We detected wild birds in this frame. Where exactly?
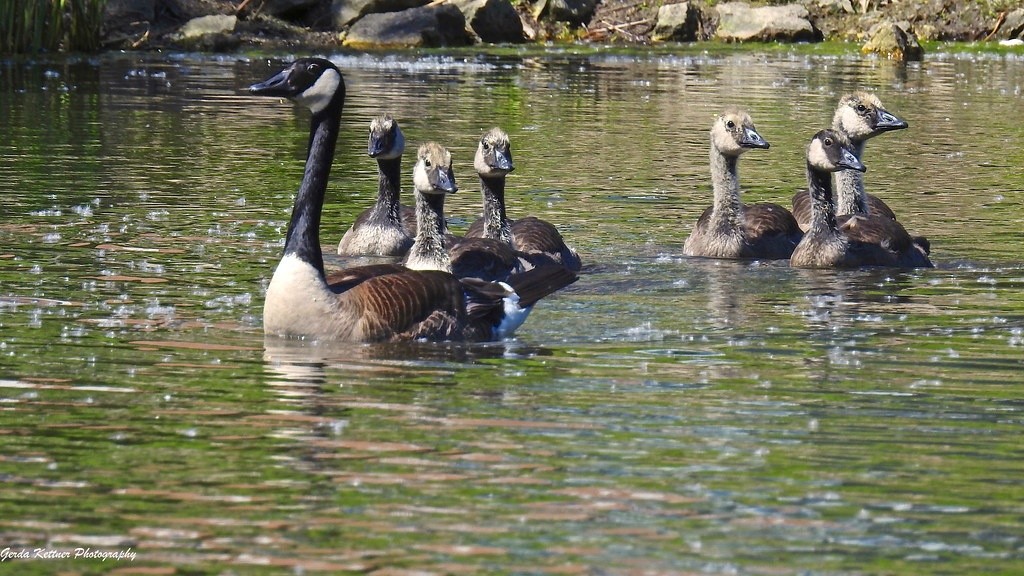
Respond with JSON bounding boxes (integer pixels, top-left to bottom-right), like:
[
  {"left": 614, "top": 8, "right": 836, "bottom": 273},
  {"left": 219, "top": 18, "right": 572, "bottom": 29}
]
[
  {"left": 682, "top": 89, "right": 937, "bottom": 270},
  {"left": 245, "top": 55, "right": 585, "bottom": 346}
]
